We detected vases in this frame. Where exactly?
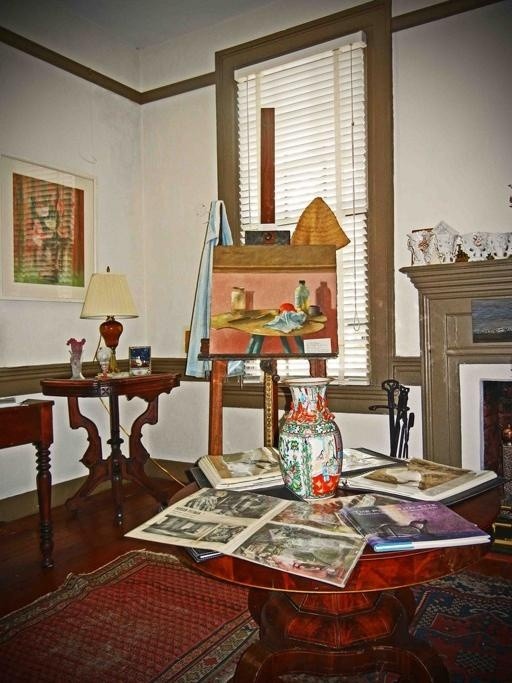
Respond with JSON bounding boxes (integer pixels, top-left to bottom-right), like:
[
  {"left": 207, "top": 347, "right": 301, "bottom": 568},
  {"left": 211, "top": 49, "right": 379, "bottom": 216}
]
[{"left": 278, "top": 377, "right": 344, "bottom": 502}]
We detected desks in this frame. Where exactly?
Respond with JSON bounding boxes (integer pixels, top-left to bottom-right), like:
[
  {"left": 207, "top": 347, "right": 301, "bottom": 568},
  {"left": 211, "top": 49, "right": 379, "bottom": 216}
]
[
  {"left": 167, "top": 479, "right": 502, "bottom": 682},
  {"left": 1, "top": 398, "right": 55, "bottom": 568},
  {"left": 39, "top": 372, "right": 183, "bottom": 526}
]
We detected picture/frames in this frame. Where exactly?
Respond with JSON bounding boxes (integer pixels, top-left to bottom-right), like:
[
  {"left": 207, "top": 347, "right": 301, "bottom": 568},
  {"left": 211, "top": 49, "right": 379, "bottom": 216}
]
[{"left": 1, "top": 152, "right": 98, "bottom": 303}]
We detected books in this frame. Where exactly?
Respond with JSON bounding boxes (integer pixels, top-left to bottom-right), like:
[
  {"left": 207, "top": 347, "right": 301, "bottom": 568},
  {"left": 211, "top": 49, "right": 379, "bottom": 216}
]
[
  {"left": 346, "top": 499, "right": 492, "bottom": 553},
  {"left": 309, "top": 493, "right": 414, "bottom": 507},
  {"left": 340, "top": 456, "right": 509, "bottom": 507},
  {"left": 188, "top": 444, "right": 396, "bottom": 491},
  {"left": 122, "top": 487, "right": 373, "bottom": 590},
  {"left": 157, "top": 504, "right": 222, "bottom": 563}
]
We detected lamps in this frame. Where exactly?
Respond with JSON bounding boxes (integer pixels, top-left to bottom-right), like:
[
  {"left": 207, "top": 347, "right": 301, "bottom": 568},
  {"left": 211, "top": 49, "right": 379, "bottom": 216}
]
[{"left": 80, "top": 266, "right": 140, "bottom": 376}]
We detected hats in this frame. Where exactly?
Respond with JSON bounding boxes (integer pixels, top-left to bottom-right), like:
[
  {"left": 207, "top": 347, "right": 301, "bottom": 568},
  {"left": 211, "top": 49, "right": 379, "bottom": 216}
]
[{"left": 289, "top": 197, "right": 351, "bottom": 249}]
